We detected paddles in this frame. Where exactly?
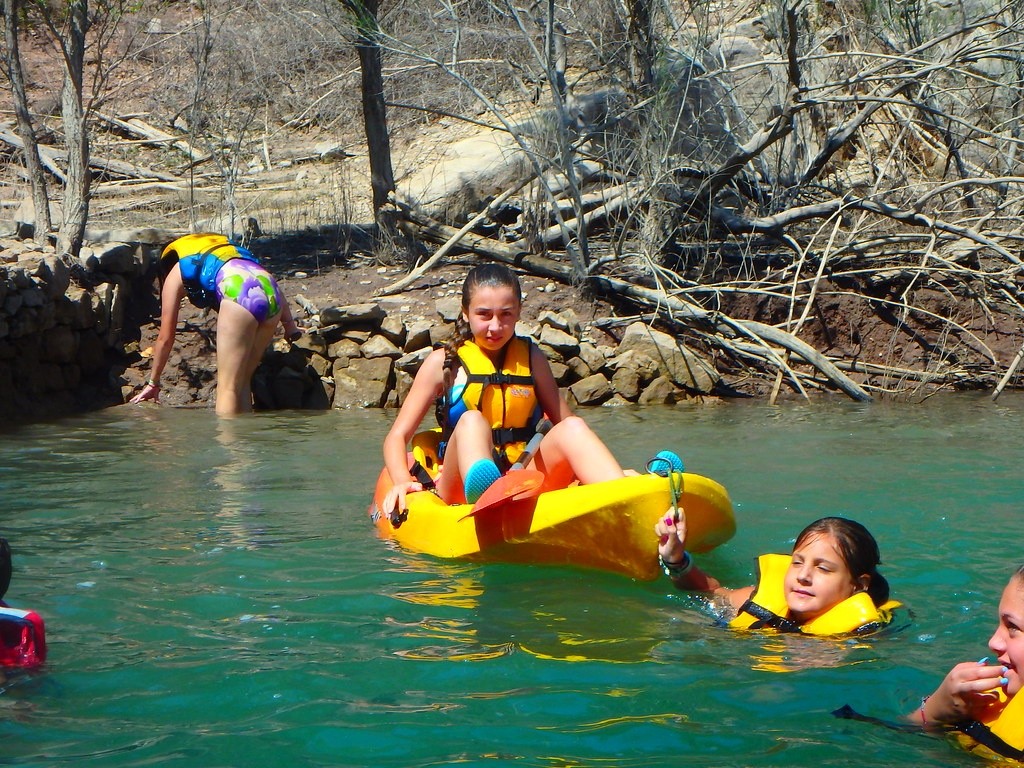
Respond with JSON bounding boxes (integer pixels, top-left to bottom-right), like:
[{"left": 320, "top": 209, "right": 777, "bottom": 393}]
[{"left": 456, "top": 418, "right": 552, "bottom": 526}]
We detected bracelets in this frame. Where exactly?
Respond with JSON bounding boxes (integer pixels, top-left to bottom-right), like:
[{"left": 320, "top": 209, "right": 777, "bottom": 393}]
[
  {"left": 658, "top": 551, "right": 695, "bottom": 578},
  {"left": 148, "top": 379, "right": 160, "bottom": 389},
  {"left": 279, "top": 316, "right": 293, "bottom": 326},
  {"left": 920, "top": 695, "right": 941, "bottom": 727}
]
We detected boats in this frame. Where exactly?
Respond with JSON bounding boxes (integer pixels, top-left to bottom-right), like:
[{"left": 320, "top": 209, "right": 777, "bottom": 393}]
[{"left": 370, "top": 428, "right": 739, "bottom": 580}]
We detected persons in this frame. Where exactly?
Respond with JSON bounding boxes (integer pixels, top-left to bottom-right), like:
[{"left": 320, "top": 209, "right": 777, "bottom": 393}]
[
  {"left": 382, "top": 263, "right": 628, "bottom": 514},
  {"left": 128, "top": 231, "right": 305, "bottom": 419},
  {"left": 654, "top": 505, "right": 893, "bottom": 639},
  {"left": 905, "top": 566, "right": 1023, "bottom": 767}
]
